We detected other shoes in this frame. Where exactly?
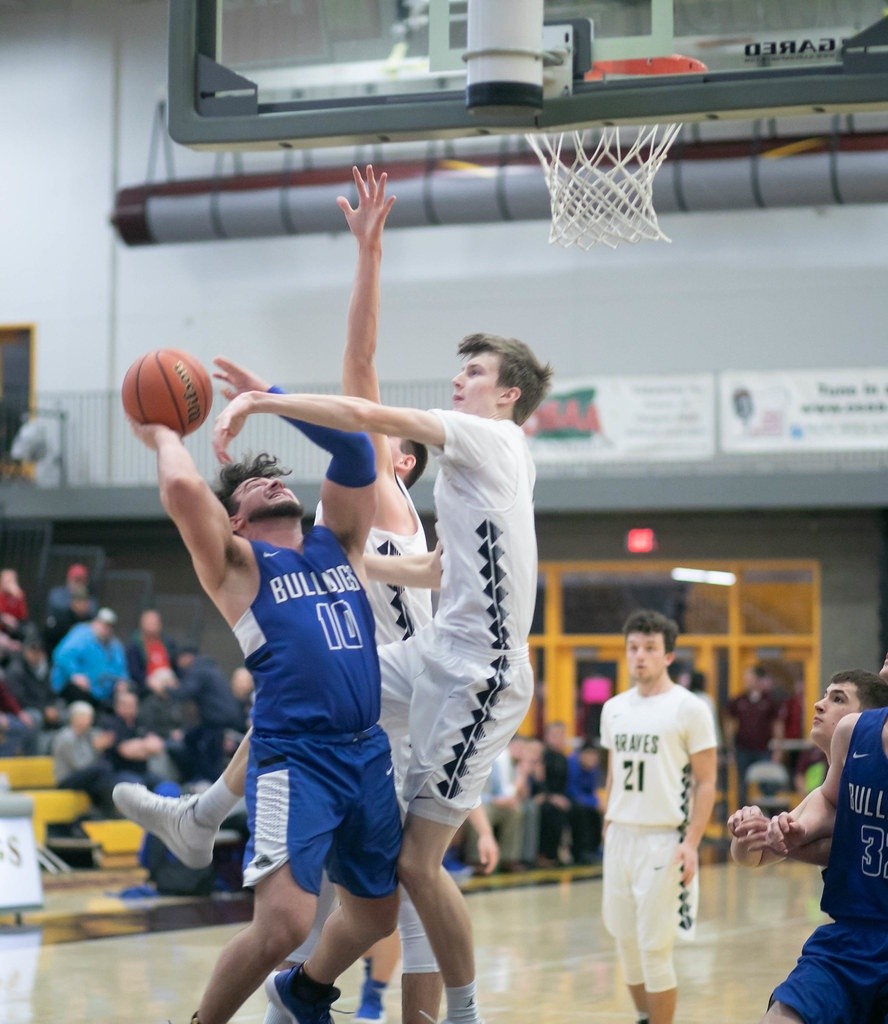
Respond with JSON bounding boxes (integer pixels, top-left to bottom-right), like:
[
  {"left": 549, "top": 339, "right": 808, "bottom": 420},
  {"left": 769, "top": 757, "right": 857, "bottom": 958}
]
[
  {"left": 440, "top": 855, "right": 477, "bottom": 877},
  {"left": 355, "top": 981, "right": 388, "bottom": 1022}
]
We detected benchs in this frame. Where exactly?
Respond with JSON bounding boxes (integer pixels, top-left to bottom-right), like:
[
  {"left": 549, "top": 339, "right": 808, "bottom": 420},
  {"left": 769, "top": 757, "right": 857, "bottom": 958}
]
[{"left": 0, "top": 731, "right": 240, "bottom": 891}]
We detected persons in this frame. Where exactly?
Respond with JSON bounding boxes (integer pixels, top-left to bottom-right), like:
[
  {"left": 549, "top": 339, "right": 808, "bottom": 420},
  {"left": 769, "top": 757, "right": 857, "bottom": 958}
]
[
  {"left": 600, "top": 609, "right": 719, "bottom": 1024},
  {"left": 268, "top": 166, "right": 524, "bottom": 1023},
  {"left": 137, "top": 355, "right": 408, "bottom": 1023},
  {"left": 727, "top": 654, "right": 888, "bottom": 1024},
  {"left": 113, "top": 332, "right": 552, "bottom": 1024},
  {"left": 729, "top": 667, "right": 791, "bottom": 820},
  {"left": 1, "top": 566, "right": 607, "bottom": 900}
]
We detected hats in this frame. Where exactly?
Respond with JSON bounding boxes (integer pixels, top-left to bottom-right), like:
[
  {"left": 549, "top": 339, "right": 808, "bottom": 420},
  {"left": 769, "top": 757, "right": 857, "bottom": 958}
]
[
  {"left": 98, "top": 608, "right": 117, "bottom": 625},
  {"left": 66, "top": 564, "right": 87, "bottom": 581}
]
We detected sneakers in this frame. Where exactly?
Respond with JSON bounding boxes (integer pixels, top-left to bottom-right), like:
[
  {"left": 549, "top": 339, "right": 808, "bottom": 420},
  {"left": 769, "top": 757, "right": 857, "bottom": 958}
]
[
  {"left": 112, "top": 782, "right": 218, "bottom": 868},
  {"left": 264, "top": 960, "right": 341, "bottom": 1024}
]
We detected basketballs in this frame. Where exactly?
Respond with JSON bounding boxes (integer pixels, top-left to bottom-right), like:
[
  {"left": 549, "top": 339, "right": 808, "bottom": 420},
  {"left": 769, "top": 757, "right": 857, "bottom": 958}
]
[{"left": 120, "top": 345, "right": 216, "bottom": 439}]
